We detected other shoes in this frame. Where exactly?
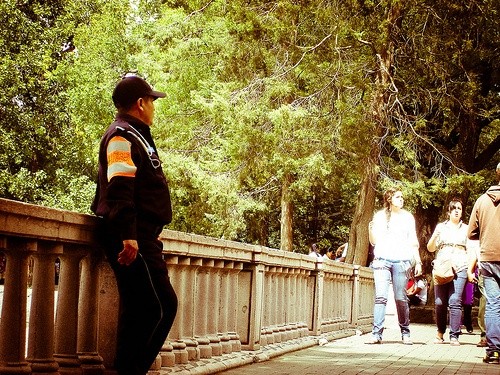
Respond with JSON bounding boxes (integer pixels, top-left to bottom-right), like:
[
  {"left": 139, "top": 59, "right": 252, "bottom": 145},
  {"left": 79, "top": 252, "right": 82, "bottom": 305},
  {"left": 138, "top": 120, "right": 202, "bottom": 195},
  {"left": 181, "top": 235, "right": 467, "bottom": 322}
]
[
  {"left": 364, "top": 337, "right": 380, "bottom": 344},
  {"left": 402, "top": 334, "right": 412, "bottom": 344},
  {"left": 436, "top": 337, "right": 444, "bottom": 343},
  {"left": 483, "top": 354, "right": 498, "bottom": 363},
  {"left": 469, "top": 330, "right": 472, "bottom": 334},
  {"left": 450, "top": 335, "right": 460, "bottom": 346},
  {"left": 477, "top": 339, "right": 488, "bottom": 346}
]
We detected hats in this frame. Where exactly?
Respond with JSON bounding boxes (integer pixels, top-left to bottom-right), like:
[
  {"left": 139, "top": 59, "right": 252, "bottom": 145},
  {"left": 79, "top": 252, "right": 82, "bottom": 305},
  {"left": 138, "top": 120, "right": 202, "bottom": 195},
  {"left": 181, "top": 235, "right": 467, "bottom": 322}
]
[
  {"left": 112, "top": 77, "right": 165, "bottom": 101},
  {"left": 311, "top": 244, "right": 319, "bottom": 250}
]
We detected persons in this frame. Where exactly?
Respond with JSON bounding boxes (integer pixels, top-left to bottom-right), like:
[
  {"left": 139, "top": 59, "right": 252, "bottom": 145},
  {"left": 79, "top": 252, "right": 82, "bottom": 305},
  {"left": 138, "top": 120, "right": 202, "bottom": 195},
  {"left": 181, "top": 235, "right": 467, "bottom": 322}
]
[
  {"left": 406, "top": 267, "right": 427, "bottom": 321},
  {"left": 426, "top": 198, "right": 469, "bottom": 346},
  {"left": 363, "top": 186, "right": 429, "bottom": 344},
  {"left": 467, "top": 173, "right": 500, "bottom": 365},
  {"left": 461, "top": 222, "right": 489, "bottom": 346},
  {"left": 90, "top": 76, "right": 178, "bottom": 375},
  {"left": 308, "top": 242, "right": 349, "bottom": 262}
]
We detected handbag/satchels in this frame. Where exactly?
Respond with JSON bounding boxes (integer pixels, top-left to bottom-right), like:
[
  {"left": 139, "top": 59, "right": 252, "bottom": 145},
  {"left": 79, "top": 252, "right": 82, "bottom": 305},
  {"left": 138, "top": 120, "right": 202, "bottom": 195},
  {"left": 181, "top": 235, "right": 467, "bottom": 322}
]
[{"left": 433, "top": 263, "right": 454, "bottom": 285}]
